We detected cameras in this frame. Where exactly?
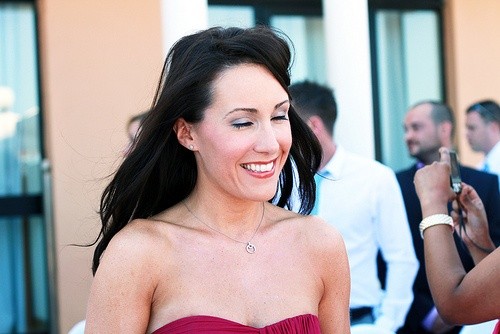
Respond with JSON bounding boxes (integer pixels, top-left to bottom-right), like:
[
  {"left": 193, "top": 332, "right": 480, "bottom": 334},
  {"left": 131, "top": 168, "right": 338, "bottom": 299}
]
[{"left": 439, "top": 152, "right": 462, "bottom": 194}]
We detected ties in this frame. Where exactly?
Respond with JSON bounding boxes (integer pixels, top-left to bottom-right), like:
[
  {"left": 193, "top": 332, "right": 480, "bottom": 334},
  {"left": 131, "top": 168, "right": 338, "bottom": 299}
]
[{"left": 310, "top": 171, "right": 329, "bottom": 216}]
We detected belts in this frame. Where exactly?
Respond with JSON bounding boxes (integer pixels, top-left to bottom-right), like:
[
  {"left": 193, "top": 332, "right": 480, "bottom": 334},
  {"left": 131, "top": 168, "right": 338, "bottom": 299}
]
[{"left": 350, "top": 306, "right": 372, "bottom": 326}]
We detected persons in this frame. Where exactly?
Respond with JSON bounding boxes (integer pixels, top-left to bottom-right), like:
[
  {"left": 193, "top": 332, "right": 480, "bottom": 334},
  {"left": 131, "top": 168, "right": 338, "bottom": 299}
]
[
  {"left": 271, "top": 80, "right": 420, "bottom": 334},
  {"left": 66, "top": 23, "right": 351, "bottom": 334}
]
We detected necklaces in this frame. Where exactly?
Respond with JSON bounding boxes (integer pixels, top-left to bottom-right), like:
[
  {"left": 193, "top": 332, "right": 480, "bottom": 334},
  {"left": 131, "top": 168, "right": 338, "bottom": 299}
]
[
  {"left": 376, "top": 101, "right": 500, "bottom": 333},
  {"left": 183, "top": 198, "right": 265, "bottom": 254}
]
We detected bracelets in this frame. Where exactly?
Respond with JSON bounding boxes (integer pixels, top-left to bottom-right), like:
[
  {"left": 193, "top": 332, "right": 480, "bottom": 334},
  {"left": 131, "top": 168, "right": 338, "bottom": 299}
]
[{"left": 420, "top": 213, "right": 453, "bottom": 240}]
